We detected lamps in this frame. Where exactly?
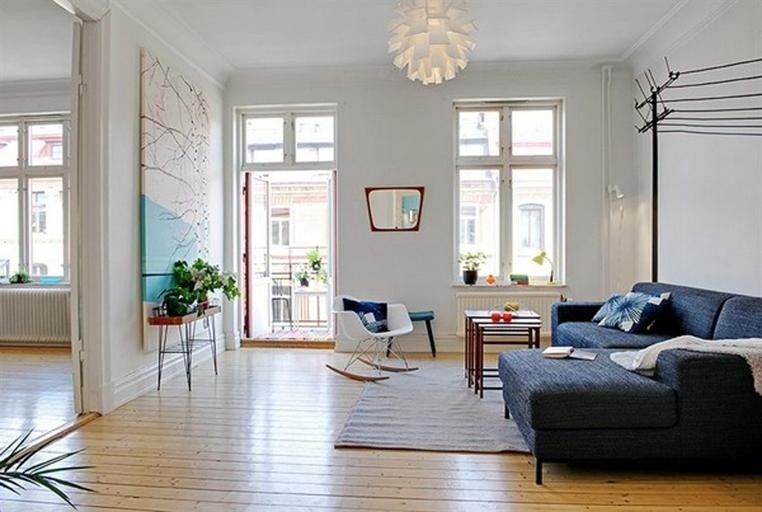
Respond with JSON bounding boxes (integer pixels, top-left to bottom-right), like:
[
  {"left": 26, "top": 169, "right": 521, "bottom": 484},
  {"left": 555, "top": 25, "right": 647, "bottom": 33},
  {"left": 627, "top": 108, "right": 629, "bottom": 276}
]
[
  {"left": 388, "top": 0, "right": 478, "bottom": 87},
  {"left": 532, "top": 250, "right": 555, "bottom": 284}
]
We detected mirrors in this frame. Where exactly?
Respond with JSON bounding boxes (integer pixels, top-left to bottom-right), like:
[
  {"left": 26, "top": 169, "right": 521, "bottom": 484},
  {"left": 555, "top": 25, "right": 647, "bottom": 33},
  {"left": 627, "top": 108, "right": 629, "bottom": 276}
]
[{"left": 364, "top": 186, "right": 425, "bottom": 232}]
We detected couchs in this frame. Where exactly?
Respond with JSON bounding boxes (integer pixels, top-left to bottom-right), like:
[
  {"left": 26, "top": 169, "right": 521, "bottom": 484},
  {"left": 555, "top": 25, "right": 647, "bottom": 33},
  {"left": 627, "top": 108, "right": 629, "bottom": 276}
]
[{"left": 550, "top": 282, "right": 762, "bottom": 474}]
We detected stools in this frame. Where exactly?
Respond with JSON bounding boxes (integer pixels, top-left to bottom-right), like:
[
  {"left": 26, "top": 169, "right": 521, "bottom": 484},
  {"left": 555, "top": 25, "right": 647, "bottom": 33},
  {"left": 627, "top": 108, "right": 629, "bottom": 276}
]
[
  {"left": 386, "top": 310, "right": 437, "bottom": 358},
  {"left": 495, "top": 347, "right": 678, "bottom": 488},
  {"left": 272, "top": 294, "right": 292, "bottom": 330}
]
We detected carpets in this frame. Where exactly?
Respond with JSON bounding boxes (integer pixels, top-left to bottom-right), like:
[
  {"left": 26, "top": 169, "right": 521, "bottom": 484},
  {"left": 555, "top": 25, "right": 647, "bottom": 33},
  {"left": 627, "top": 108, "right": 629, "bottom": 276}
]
[{"left": 335, "top": 356, "right": 532, "bottom": 456}]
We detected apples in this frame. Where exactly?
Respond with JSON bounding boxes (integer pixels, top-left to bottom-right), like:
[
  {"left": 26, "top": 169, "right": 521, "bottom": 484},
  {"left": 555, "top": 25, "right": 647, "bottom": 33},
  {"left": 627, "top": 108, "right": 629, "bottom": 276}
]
[
  {"left": 491, "top": 313, "right": 500, "bottom": 321},
  {"left": 502, "top": 313, "right": 513, "bottom": 322}
]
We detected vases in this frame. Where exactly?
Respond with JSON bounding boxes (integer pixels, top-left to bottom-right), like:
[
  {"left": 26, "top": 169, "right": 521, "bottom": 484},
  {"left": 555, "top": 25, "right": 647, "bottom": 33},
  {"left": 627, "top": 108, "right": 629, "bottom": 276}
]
[{"left": 195, "top": 300, "right": 209, "bottom": 310}]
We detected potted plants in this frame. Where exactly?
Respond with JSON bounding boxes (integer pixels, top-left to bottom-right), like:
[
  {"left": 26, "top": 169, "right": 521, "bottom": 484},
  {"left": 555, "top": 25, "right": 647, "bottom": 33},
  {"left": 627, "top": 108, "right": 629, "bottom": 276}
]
[
  {"left": 458, "top": 249, "right": 487, "bottom": 285},
  {"left": 294, "top": 250, "right": 322, "bottom": 287},
  {"left": 157, "top": 288, "right": 196, "bottom": 317}
]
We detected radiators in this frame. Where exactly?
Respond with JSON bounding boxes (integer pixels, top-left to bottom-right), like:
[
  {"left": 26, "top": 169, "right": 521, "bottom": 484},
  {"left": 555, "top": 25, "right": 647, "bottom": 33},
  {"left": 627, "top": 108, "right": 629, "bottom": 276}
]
[
  {"left": 455, "top": 291, "right": 563, "bottom": 339},
  {"left": 0, "top": 287, "right": 71, "bottom": 344}
]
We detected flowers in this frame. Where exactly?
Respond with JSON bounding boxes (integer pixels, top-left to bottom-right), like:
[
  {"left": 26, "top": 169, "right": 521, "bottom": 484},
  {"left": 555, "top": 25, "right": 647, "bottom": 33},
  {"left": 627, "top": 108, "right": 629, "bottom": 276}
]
[{"left": 173, "top": 258, "right": 241, "bottom": 302}]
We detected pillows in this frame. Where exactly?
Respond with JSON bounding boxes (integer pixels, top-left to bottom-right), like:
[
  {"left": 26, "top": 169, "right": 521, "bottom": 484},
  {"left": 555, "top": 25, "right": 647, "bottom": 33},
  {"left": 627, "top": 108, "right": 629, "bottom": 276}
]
[
  {"left": 591, "top": 290, "right": 672, "bottom": 335},
  {"left": 343, "top": 298, "right": 389, "bottom": 332}
]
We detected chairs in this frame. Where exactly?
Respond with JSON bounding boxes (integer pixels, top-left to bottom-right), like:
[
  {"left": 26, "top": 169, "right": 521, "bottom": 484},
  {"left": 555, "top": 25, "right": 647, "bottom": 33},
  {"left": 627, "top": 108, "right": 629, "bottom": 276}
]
[{"left": 326, "top": 294, "right": 419, "bottom": 380}]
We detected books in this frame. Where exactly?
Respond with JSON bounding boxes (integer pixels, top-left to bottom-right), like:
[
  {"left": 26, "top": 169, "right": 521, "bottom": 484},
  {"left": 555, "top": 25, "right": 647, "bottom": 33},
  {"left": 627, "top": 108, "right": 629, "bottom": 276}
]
[{"left": 541, "top": 345, "right": 598, "bottom": 360}]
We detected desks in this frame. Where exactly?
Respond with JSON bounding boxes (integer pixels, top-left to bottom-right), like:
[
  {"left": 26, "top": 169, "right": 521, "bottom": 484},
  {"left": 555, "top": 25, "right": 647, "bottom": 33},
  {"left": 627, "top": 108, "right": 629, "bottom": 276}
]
[
  {"left": 149, "top": 305, "right": 222, "bottom": 391},
  {"left": 294, "top": 287, "right": 329, "bottom": 332}
]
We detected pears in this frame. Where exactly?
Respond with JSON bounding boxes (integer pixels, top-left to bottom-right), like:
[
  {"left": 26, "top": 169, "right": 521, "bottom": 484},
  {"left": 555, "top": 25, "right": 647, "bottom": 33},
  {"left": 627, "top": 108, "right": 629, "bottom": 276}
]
[{"left": 504, "top": 302, "right": 520, "bottom": 312}]
